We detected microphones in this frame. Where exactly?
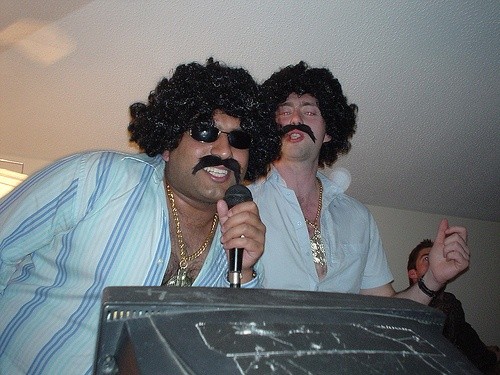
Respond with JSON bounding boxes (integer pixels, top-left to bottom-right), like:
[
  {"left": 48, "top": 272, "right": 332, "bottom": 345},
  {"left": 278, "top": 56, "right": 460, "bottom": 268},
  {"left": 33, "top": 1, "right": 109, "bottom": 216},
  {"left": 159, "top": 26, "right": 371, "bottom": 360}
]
[{"left": 222, "top": 184, "right": 251, "bottom": 289}]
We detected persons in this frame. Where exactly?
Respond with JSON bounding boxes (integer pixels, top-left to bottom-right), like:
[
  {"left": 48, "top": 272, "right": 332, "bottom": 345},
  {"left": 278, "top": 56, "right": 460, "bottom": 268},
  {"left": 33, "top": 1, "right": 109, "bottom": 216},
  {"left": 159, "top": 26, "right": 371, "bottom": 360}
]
[
  {"left": 406, "top": 237, "right": 468, "bottom": 322},
  {"left": 239, "top": 61, "right": 472, "bottom": 306},
  {"left": 1, "top": 59, "right": 283, "bottom": 374}
]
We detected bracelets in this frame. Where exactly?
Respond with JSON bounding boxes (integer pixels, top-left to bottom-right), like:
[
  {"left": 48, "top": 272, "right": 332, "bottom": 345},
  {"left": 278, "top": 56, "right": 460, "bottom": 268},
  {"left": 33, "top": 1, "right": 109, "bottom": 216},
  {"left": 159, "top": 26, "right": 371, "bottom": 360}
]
[{"left": 415, "top": 274, "right": 436, "bottom": 298}]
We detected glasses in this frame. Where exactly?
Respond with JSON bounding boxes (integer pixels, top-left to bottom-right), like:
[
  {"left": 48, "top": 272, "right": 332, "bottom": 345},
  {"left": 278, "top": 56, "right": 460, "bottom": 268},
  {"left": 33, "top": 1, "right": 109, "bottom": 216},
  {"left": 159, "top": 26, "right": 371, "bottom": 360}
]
[{"left": 189, "top": 122, "right": 251, "bottom": 149}]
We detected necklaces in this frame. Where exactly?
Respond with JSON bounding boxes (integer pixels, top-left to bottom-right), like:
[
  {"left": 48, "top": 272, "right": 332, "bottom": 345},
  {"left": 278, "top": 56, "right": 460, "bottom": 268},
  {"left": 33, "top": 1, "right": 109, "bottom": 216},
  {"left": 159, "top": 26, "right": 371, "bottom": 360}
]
[
  {"left": 163, "top": 183, "right": 217, "bottom": 287},
  {"left": 302, "top": 175, "right": 326, "bottom": 268}
]
